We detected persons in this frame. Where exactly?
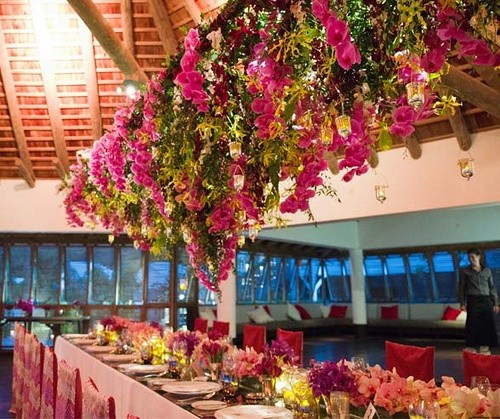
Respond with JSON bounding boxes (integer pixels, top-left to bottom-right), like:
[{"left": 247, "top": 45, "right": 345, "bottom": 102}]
[{"left": 459, "top": 248, "right": 498, "bottom": 354}]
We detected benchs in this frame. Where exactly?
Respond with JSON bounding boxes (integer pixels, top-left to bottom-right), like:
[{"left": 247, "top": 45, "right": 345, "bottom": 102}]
[
  {"left": 199, "top": 302, "right": 339, "bottom": 345},
  {"left": 329, "top": 303, "right": 468, "bottom": 344}
]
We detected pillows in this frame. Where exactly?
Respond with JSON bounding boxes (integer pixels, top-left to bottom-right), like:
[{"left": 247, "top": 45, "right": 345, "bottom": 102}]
[
  {"left": 287, "top": 303, "right": 313, "bottom": 321},
  {"left": 437, "top": 306, "right": 467, "bottom": 321},
  {"left": 381, "top": 305, "right": 399, "bottom": 320},
  {"left": 320, "top": 304, "right": 348, "bottom": 319},
  {"left": 246, "top": 306, "right": 274, "bottom": 324}
]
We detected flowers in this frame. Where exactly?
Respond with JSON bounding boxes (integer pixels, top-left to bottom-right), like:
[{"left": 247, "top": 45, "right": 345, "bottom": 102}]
[
  {"left": 92, "top": 316, "right": 500, "bottom": 419},
  {"left": 306, "top": 358, "right": 500, "bottom": 419},
  {"left": 64, "top": 0, "right": 500, "bottom": 298}
]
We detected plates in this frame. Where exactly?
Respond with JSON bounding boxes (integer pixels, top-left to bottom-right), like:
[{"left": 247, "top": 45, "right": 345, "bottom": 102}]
[
  {"left": 62, "top": 332, "right": 118, "bottom": 353},
  {"left": 191, "top": 400, "right": 227, "bottom": 410},
  {"left": 104, "top": 354, "right": 133, "bottom": 361},
  {"left": 214, "top": 405, "right": 294, "bottom": 419},
  {"left": 160, "top": 381, "right": 220, "bottom": 398},
  {"left": 150, "top": 377, "right": 176, "bottom": 385},
  {"left": 119, "top": 363, "right": 165, "bottom": 373}
]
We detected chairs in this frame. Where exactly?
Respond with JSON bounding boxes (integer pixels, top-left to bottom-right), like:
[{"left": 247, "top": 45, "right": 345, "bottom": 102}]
[
  {"left": 8, "top": 321, "right": 144, "bottom": 419},
  {"left": 194, "top": 317, "right": 500, "bottom": 393}
]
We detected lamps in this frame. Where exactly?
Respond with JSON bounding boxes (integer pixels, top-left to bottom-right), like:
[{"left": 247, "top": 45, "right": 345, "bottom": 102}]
[
  {"left": 375, "top": 185, "right": 389, "bottom": 204},
  {"left": 457, "top": 158, "right": 474, "bottom": 182}
]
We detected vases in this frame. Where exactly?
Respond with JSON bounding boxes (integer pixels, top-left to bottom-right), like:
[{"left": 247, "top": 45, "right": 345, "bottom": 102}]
[
  {"left": 220, "top": 369, "right": 239, "bottom": 397},
  {"left": 209, "top": 363, "right": 223, "bottom": 381},
  {"left": 169, "top": 355, "right": 182, "bottom": 375},
  {"left": 262, "top": 375, "right": 278, "bottom": 404},
  {"left": 330, "top": 391, "right": 349, "bottom": 419}
]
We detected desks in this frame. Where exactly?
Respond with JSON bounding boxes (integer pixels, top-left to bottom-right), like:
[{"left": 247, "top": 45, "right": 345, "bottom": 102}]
[{"left": 54, "top": 334, "right": 325, "bottom": 419}]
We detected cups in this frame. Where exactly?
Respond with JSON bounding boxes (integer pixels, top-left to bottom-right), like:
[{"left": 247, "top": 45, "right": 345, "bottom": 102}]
[
  {"left": 261, "top": 374, "right": 276, "bottom": 400},
  {"left": 167, "top": 356, "right": 179, "bottom": 378},
  {"left": 140, "top": 345, "right": 153, "bottom": 365},
  {"left": 408, "top": 397, "right": 439, "bottom": 419},
  {"left": 122, "top": 334, "right": 132, "bottom": 353},
  {"left": 293, "top": 393, "right": 319, "bottom": 419},
  {"left": 92, "top": 320, "right": 117, "bottom": 346},
  {"left": 221, "top": 371, "right": 238, "bottom": 406},
  {"left": 330, "top": 392, "right": 350, "bottom": 419},
  {"left": 470, "top": 375, "right": 491, "bottom": 397}
]
